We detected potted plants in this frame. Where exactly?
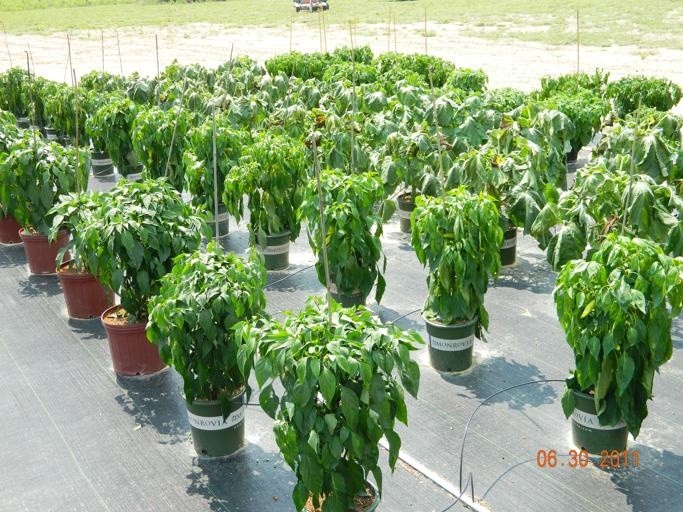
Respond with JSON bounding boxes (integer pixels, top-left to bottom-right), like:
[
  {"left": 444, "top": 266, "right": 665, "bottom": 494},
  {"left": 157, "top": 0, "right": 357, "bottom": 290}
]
[
  {"left": 552, "top": 227, "right": 683, "bottom": 458},
  {"left": 103, "top": 101, "right": 145, "bottom": 183},
  {"left": 44, "top": 190, "right": 122, "bottom": 321},
  {"left": 145, "top": 240, "right": 272, "bottom": 460},
  {"left": 543, "top": 86, "right": 610, "bottom": 163},
  {"left": 0, "top": 131, "right": 92, "bottom": 274},
  {"left": 8, "top": 70, "right": 30, "bottom": 129},
  {"left": 45, "top": 95, "right": 65, "bottom": 142},
  {"left": 368, "top": 87, "right": 457, "bottom": 234},
  {"left": 77, "top": 177, "right": 214, "bottom": 378},
  {"left": 230, "top": 295, "right": 424, "bottom": 508},
  {"left": 131, "top": 110, "right": 183, "bottom": 205},
  {"left": 85, "top": 112, "right": 115, "bottom": 178},
  {"left": 0, "top": 127, "right": 44, "bottom": 246},
  {"left": 410, "top": 185, "right": 503, "bottom": 374},
  {"left": 295, "top": 168, "right": 396, "bottom": 316},
  {"left": 182, "top": 115, "right": 246, "bottom": 240},
  {"left": 445, "top": 129, "right": 547, "bottom": 265},
  {"left": 222, "top": 135, "right": 310, "bottom": 271}
]
